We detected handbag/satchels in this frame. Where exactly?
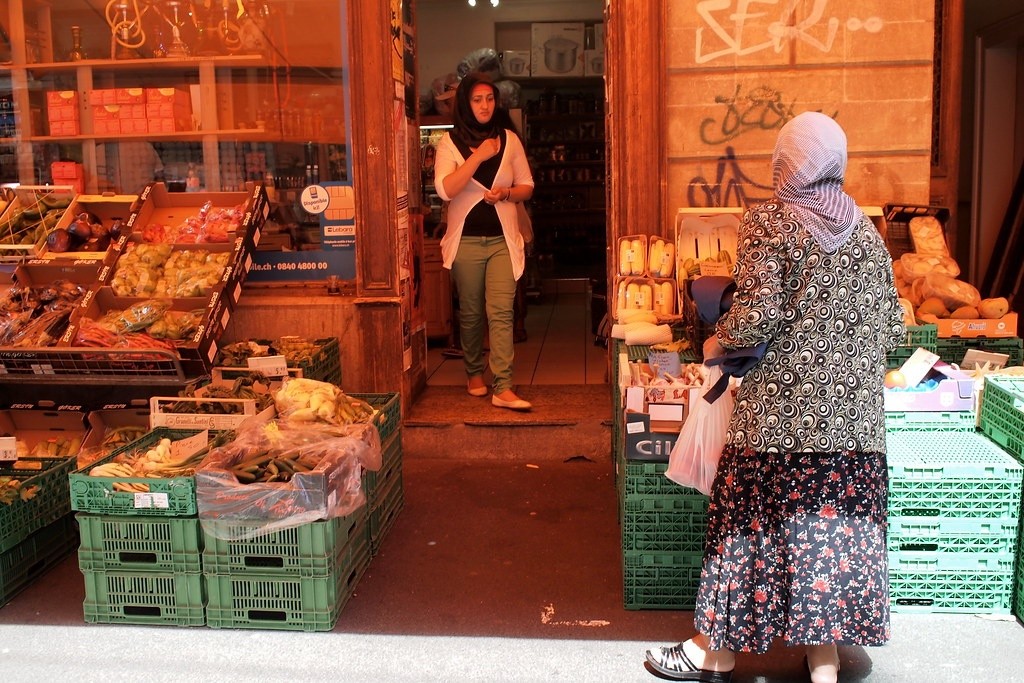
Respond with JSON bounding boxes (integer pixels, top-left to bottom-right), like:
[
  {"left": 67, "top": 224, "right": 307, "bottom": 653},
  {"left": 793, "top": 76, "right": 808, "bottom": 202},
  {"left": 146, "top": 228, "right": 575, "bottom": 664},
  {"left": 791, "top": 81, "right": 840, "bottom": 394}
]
[
  {"left": 691, "top": 276, "right": 770, "bottom": 404},
  {"left": 664, "top": 364, "right": 734, "bottom": 496}
]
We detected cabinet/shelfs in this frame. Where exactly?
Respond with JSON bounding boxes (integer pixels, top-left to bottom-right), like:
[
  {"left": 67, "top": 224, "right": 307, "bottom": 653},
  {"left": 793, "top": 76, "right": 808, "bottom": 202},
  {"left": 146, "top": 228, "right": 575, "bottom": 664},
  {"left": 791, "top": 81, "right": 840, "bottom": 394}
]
[
  {"left": 0, "top": 0, "right": 266, "bottom": 195},
  {"left": 504, "top": 77, "right": 606, "bottom": 216}
]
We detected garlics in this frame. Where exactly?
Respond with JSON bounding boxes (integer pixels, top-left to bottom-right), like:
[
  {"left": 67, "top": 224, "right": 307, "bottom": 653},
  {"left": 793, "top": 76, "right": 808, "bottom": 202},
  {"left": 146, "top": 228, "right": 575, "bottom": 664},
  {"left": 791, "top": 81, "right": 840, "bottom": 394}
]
[{"left": 623, "top": 359, "right": 703, "bottom": 386}]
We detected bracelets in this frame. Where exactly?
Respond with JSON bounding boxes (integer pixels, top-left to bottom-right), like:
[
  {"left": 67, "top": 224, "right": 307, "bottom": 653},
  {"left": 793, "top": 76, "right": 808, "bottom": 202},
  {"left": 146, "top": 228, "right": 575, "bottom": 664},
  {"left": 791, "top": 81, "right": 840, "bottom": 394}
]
[{"left": 506, "top": 188, "right": 510, "bottom": 200}]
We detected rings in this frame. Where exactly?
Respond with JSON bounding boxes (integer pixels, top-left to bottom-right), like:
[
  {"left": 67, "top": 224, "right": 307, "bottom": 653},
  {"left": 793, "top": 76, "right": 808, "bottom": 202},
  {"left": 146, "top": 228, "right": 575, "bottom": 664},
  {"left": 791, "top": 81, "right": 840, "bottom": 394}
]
[{"left": 487, "top": 201, "right": 490, "bottom": 203}]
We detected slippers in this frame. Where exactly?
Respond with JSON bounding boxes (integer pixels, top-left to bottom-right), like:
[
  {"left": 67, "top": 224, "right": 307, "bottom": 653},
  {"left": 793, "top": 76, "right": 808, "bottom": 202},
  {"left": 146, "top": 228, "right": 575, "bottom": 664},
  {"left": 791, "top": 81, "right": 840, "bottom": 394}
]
[
  {"left": 803, "top": 654, "right": 840, "bottom": 683},
  {"left": 645, "top": 639, "right": 735, "bottom": 683}
]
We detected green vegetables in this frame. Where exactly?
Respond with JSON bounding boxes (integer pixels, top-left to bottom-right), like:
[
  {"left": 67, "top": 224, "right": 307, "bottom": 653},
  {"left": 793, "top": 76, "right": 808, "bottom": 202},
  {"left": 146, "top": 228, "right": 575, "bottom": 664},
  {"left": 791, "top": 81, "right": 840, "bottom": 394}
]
[{"left": 157, "top": 338, "right": 279, "bottom": 414}]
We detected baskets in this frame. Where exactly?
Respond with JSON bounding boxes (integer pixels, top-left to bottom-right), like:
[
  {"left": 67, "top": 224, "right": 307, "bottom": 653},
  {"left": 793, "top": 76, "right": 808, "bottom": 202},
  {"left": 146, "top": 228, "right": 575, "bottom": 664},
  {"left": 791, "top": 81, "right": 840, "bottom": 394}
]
[
  {"left": 883, "top": 203, "right": 952, "bottom": 280},
  {"left": 68, "top": 425, "right": 236, "bottom": 515},
  {"left": 345, "top": 392, "right": 400, "bottom": 446}
]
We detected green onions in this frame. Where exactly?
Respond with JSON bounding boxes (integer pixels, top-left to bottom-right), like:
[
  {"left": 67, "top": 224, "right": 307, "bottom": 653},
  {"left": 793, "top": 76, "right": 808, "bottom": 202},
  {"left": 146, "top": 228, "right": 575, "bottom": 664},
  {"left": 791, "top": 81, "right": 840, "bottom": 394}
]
[{"left": 86, "top": 427, "right": 239, "bottom": 494}]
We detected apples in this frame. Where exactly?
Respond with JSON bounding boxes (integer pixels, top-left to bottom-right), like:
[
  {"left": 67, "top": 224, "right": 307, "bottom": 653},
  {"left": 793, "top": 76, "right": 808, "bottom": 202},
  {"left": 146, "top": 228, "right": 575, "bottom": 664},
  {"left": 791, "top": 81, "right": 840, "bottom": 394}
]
[{"left": 883, "top": 371, "right": 906, "bottom": 387}]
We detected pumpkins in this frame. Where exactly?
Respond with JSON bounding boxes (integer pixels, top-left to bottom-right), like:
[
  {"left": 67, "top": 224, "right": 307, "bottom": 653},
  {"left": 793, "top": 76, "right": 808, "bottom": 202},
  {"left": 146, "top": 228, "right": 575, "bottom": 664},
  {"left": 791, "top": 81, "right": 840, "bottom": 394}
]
[{"left": 918, "top": 294, "right": 1008, "bottom": 319}]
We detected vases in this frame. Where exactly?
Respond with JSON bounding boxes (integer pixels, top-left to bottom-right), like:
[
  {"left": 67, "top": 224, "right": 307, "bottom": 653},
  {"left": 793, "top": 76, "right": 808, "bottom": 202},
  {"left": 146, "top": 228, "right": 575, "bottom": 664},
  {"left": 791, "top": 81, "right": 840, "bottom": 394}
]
[{"left": 62, "top": 25, "right": 86, "bottom": 60}]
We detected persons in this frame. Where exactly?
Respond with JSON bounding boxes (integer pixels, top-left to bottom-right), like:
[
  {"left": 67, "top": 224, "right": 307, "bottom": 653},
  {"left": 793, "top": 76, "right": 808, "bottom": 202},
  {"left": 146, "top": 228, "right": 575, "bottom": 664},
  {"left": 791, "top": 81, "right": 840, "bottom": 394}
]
[
  {"left": 434, "top": 72, "right": 534, "bottom": 409},
  {"left": 97, "top": 142, "right": 168, "bottom": 194},
  {"left": 645, "top": 111, "right": 907, "bottom": 683}
]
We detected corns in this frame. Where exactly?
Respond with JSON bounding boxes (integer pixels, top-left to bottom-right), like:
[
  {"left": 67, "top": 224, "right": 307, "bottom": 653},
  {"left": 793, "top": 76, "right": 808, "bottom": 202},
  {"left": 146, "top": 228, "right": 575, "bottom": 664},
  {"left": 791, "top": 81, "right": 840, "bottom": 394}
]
[{"left": 618, "top": 239, "right": 675, "bottom": 317}]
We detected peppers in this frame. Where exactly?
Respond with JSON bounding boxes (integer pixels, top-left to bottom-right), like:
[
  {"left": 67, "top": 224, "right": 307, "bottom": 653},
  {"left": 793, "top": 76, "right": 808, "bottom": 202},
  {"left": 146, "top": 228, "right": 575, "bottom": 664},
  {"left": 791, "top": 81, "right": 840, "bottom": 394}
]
[
  {"left": 110, "top": 244, "right": 230, "bottom": 296},
  {"left": 0, "top": 193, "right": 73, "bottom": 255},
  {"left": 73, "top": 323, "right": 180, "bottom": 367}
]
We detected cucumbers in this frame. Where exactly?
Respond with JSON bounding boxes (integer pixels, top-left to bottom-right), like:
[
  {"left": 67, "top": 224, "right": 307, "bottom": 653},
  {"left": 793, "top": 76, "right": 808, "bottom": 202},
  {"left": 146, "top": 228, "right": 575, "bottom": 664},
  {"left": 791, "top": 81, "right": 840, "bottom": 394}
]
[
  {"left": 678, "top": 250, "right": 734, "bottom": 291},
  {"left": 228, "top": 448, "right": 311, "bottom": 484}
]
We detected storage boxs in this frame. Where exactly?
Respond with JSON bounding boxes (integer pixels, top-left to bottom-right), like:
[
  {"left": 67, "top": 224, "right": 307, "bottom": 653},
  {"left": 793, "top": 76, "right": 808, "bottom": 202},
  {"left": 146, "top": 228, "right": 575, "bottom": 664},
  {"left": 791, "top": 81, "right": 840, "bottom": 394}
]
[
  {"left": 47, "top": 88, "right": 177, "bottom": 138},
  {"left": 502, "top": 50, "right": 530, "bottom": 77},
  {"left": 0, "top": 162, "right": 404, "bottom": 632},
  {"left": 584, "top": 49, "right": 606, "bottom": 77},
  {"left": 531, "top": 22, "right": 585, "bottom": 77},
  {"left": 611, "top": 201, "right": 1024, "bottom": 625},
  {"left": 594, "top": 23, "right": 604, "bottom": 49}
]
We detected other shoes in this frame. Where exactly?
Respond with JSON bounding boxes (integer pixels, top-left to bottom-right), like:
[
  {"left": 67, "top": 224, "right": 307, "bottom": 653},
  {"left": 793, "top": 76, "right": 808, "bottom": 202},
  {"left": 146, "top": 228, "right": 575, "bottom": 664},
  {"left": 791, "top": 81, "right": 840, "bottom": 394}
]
[
  {"left": 492, "top": 394, "right": 531, "bottom": 409},
  {"left": 440, "top": 344, "right": 485, "bottom": 356},
  {"left": 513, "top": 329, "right": 528, "bottom": 344},
  {"left": 467, "top": 378, "right": 487, "bottom": 396}
]
